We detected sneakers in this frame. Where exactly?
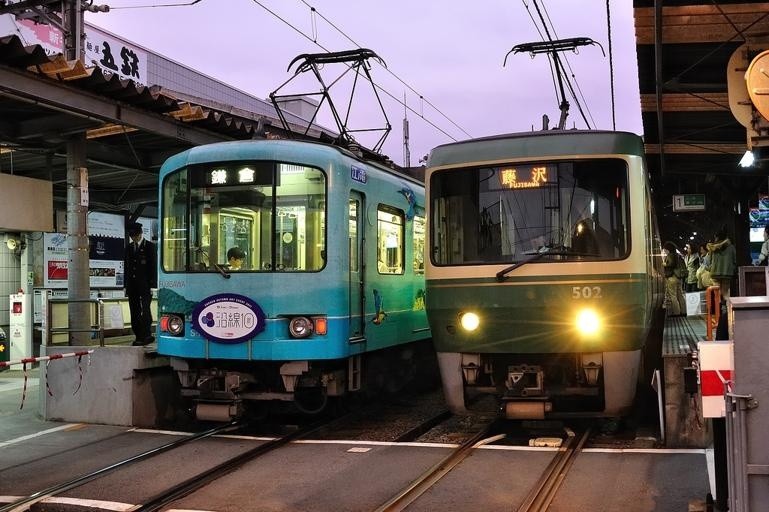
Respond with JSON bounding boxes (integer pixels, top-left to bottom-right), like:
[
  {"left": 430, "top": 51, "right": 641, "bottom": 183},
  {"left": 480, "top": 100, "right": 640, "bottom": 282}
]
[{"left": 132, "top": 335, "right": 154, "bottom": 346}]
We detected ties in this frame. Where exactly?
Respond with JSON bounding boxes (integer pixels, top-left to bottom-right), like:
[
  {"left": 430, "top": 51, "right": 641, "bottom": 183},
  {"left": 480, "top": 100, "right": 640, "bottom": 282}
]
[{"left": 135, "top": 242, "right": 138, "bottom": 251}]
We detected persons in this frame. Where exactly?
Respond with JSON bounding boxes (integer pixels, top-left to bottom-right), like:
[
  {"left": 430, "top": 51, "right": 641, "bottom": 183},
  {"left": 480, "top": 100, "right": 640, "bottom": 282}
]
[
  {"left": 224, "top": 247, "right": 245, "bottom": 271},
  {"left": 121, "top": 223, "right": 158, "bottom": 346},
  {"left": 664, "top": 224, "right": 768, "bottom": 317}
]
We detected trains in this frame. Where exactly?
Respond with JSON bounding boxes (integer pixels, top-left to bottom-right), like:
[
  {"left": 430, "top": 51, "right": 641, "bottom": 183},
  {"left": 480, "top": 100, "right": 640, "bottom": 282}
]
[
  {"left": 414, "top": 128, "right": 668, "bottom": 431},
  {"left": 151, "top": 137, "right": 433, "bottom": 425}
]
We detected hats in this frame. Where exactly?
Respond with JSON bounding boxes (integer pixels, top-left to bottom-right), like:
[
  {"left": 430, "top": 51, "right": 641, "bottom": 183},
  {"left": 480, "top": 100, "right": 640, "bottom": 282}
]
[{"left": 128, "top": 223, "right": 142, "bottom": 232}]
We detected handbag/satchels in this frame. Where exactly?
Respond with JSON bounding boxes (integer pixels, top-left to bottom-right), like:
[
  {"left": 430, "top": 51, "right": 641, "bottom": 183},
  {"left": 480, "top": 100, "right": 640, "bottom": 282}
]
[{"left": 674, "top": 262, "right": 688, "bottom": 278}]
